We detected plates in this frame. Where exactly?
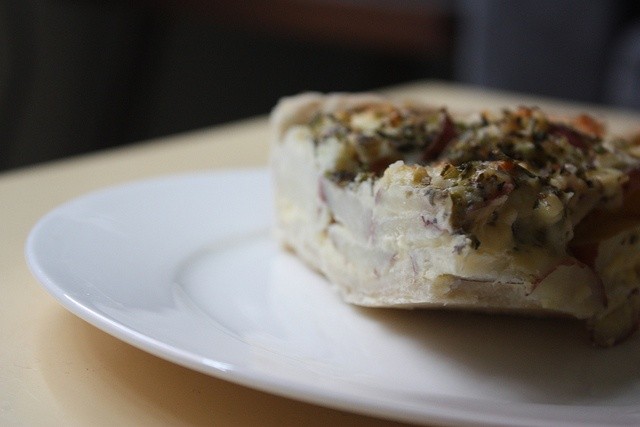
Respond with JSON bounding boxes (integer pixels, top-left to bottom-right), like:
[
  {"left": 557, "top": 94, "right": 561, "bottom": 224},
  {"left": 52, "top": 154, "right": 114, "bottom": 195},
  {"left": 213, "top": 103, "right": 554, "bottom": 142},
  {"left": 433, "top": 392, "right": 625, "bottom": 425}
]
[{"left": 26, "top": 166, "right": 635, "bottom": 426}]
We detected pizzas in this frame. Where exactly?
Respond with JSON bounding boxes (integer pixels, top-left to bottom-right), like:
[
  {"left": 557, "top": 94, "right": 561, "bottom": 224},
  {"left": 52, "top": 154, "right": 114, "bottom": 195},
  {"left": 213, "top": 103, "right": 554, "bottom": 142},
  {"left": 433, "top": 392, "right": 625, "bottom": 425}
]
[{"left": 268, "top": 92, "right": 640, "bottom": 348}]
